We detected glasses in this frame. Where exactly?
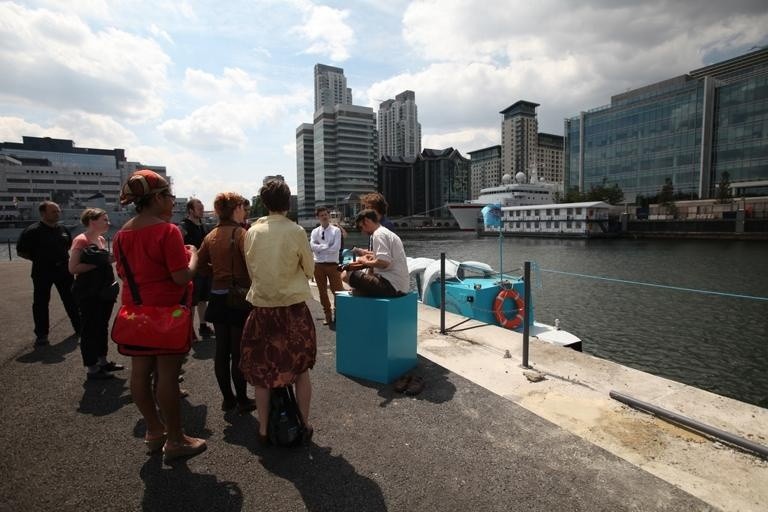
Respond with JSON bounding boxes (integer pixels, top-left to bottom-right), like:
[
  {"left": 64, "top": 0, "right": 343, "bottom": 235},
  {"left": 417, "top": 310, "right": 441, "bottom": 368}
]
[{"left": 322, "top": 231, "right": 325, "bottom": 240}]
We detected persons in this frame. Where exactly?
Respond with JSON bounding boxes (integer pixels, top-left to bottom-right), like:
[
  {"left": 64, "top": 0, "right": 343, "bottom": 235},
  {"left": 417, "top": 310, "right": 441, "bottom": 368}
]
[
  {"left": 198, "top": 194, "right": 258, "bottom": 415},
  {"left": 16, "top": 201, "right": 80, "bottom": 344},
  {"left": 68, "top": 208, "right": 124, "bottom": 380},
  {"left": 310, "top": 205, "right": 341, "bottom": 325},
  {"left": 356, "top": 191, "right": 397, "bottom": 256},
  {"left": 339, "top": 209, "right": 410, "bottom": 296},
  {"left": 179, "top": 200, "right": 215, "bottom": 342},
  {"left": 242, "top": 199, "right": 251, "bottom": 229},
  {"left": 109, "top": 169, "right": 207, "bottom": 456},
  {"left": 239, "top": 179, "right": 317, "bottom": 448}
]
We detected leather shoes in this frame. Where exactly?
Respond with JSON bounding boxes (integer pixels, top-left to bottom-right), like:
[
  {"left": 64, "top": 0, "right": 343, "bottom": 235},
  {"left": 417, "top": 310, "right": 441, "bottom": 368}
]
[
  {"left": 323, "top": 316, "right": 333, "bottom": 325},
  {"left": 392, "top": 372, "right": 426, "bottom": 396}
]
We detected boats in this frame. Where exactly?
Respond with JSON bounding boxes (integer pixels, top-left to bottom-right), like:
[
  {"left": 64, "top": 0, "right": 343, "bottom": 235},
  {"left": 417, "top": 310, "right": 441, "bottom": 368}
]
[
  {"left": 0, "top": 189, "right": 133, "bottom": 245},
  {"left": 336, "top": 249, "right": 586, "bottom": 353},
  {"left": 474, "top": 201, "right": 621, "bottom": 239}
]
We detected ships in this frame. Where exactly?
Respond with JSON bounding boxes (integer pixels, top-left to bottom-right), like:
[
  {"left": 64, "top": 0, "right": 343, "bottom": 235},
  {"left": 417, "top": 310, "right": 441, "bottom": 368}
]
[{"left": 444, "top": 153, "right": 560, "bottom": 230}]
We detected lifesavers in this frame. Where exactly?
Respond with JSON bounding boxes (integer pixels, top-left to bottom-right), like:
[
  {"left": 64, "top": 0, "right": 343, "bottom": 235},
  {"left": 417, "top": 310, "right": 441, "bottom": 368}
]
[{"left": 493, "top": 290, "right": 525, "bottom": 330}]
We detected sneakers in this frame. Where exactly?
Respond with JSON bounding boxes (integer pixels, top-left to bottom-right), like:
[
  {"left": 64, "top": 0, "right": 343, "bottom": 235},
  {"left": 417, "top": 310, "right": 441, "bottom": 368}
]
[
  {"left": 35, "top": 336, "right": 51, "bottom": 355},
  {"left": 257, "top": 433, "right": 270, "bottom": 443},
  {"left": 179, "top": 388, "right": 189, "bottom": 396},
  {"left": 222, "top": 395, "right": 256, "bottom": 412},
  {"left": 199, "top": 326, "right": 216, "bottom": 336},
  {"left": 165, "top": 434, "right": 207, "bottom": 460},
  {"left": 87, "top": 361, "right": 124, "bottom": 380},
  {"left": 303, "top": 425, "right": 313, "bottom": 438},
  {"left": 144, "top": 431, "right": 167, "bottom": 451},
  {"left": 178, "top": 375, "right": 185, "bottom": 383}
]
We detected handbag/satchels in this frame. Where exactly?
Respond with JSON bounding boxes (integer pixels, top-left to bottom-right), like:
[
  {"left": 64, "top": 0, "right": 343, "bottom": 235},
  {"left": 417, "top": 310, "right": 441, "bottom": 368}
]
[
  {"left": 111, "top": 303, "right": 193, "bottom": 357},
  {"left": 267, "top": 384, "right": 308, "bottom": 448},
  {"left": 228, "top": 283, "right": 253, "bottom": 309},
  {"left": 101, "top": 279, "right": 119, "bottom": 302}
]
